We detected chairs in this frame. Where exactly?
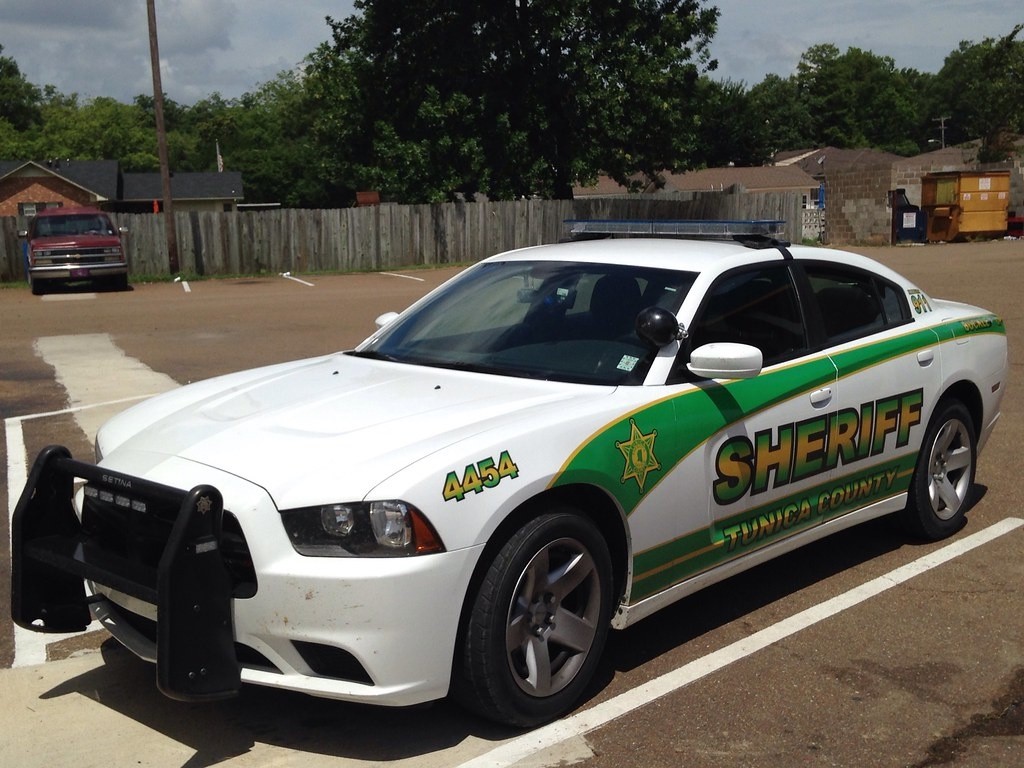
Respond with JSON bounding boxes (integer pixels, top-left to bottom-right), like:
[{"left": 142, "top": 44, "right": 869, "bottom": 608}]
[{"left": 550, "top": 274, "right": 642, "bottom": 386}]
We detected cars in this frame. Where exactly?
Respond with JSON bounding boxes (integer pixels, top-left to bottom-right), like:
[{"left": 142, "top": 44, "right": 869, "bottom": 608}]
[
  {"left": 18, "top": 204, "right": 131, "bottom": 296},
  {"left": 3, "top": 218, "right": 1015, "bottom": 728}
]
